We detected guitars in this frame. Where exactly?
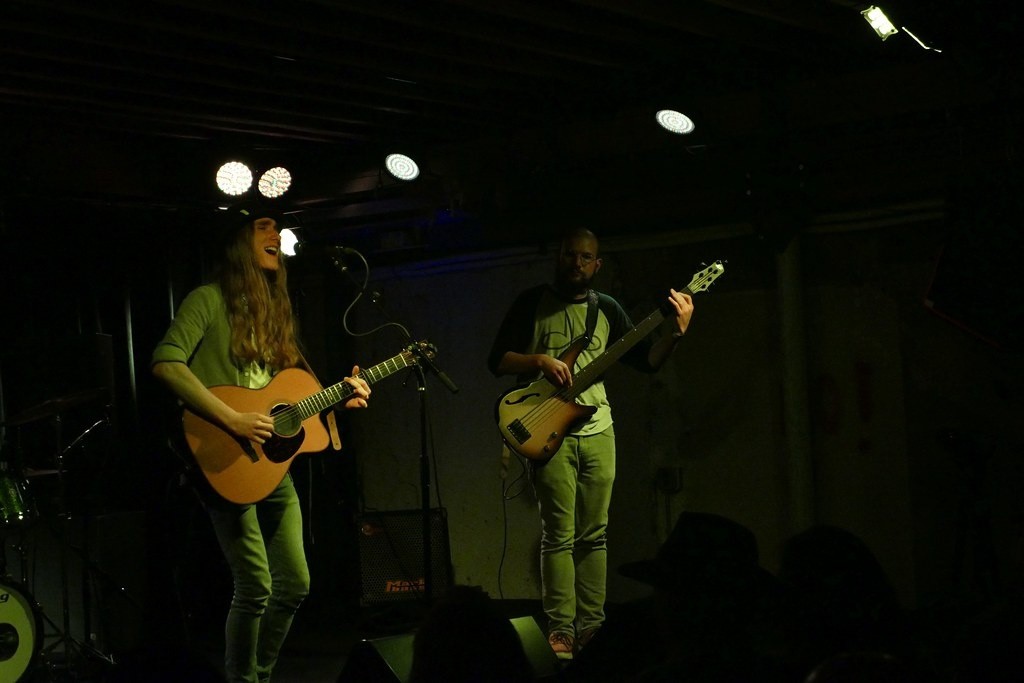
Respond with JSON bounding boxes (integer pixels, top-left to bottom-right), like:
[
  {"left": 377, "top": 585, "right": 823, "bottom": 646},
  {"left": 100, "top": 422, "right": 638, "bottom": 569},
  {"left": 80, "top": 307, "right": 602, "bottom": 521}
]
[
  {"left": 495, "top": 258, "right": 732, "bottom": 463},
  {"left": 179, "top": 340, "right": 439, "bottom": 511}
]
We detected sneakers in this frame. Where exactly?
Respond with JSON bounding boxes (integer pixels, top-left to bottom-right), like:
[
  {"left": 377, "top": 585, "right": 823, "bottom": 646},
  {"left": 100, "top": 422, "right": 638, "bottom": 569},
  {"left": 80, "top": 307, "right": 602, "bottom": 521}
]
[
  {"left": 548, "top": 632, "right": 577, "bottom": 660},
  {"left": 577, "top": 627, "right": 598, "bottom": 650}
]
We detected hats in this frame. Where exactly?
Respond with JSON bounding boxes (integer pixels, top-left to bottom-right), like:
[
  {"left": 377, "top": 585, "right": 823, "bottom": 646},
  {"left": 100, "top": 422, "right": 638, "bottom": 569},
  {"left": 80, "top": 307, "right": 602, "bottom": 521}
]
[{"left": 216, "top": 202, "right": 281, "bottom": 250}]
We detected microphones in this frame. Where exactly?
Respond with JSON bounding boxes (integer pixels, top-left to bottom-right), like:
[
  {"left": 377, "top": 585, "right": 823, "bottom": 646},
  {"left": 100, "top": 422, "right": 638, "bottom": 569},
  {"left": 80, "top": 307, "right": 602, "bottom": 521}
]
[{"left": 294, "top": 242, "right": 356, "bottom": 256}]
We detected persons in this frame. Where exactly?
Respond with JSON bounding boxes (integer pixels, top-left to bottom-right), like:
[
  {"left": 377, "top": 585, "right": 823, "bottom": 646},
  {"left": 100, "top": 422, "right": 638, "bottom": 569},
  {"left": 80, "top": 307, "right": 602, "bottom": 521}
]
[
  {"left": 486, "top": 227, "right": 695, "bottom": 660},
  {"left": 106, "top": 641, "right": 229, "bottom": 683},
  {"left": 151, "top": 205, "right": 373, "bottom": 683},
  {"left": 411, "top": 511, "right": 1024, "bottom": 683}
]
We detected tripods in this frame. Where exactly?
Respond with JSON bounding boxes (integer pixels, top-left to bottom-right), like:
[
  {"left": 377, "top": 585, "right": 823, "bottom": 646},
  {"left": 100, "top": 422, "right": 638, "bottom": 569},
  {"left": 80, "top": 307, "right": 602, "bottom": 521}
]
[{"left": 32, "top": 409, "right": 118, "bottom": 683}]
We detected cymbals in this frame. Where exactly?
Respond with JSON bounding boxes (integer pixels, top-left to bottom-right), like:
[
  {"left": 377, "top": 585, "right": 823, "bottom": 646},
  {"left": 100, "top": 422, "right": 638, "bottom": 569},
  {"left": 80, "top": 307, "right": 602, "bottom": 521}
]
[{"left": 0, "top": 387, "right": 113, "bottom": 428}]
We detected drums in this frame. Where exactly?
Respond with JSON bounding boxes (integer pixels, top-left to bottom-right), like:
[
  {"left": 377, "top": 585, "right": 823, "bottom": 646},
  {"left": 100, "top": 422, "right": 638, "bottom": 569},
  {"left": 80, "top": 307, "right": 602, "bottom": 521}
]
[
  {"left": 0, "top": 588, "right": 44, "bottom": 683},
  {"left": 0, "top": 466, "right": 33, "bottom": 528}
]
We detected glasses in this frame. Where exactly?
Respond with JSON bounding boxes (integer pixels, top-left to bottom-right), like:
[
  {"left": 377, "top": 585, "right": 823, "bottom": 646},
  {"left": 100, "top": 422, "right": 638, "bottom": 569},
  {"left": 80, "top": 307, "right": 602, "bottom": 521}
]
[{"left": 564, "top": 250, "right": 598, "bottom": 263}]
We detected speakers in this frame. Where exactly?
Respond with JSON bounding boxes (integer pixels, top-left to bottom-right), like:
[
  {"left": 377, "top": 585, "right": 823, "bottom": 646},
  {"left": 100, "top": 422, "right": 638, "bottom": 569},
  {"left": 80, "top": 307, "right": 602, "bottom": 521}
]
[
  {"left": 343, "top": 506, "right": 456, "bottom": 613},
  {"left": 88, "top": 511, "right": 190, "bottom": 661},
  {"left": 336, "top": 615, "right": 564, "bottom": 683}
]
